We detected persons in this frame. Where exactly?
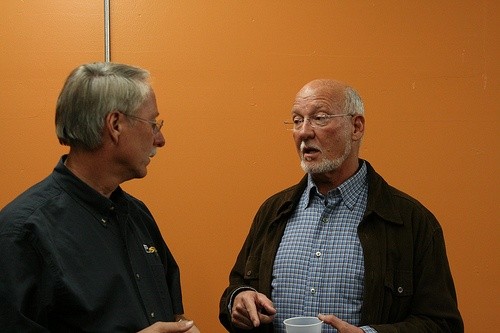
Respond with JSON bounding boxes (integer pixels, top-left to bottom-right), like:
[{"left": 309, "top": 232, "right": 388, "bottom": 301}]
[
  {"left": 219, "top": 78, "right": 465, "bottom": 333},
  {"left": 0, "top": 61, "right": 203, "bottom": 333}
]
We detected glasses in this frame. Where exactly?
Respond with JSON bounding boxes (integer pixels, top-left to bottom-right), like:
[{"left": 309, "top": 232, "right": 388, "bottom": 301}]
[
  {"left": 284, "top": 114, "right": 354, "bottom": 130},
  {"left": 118, "top": 109, "right": 164, "bottom": 135}
]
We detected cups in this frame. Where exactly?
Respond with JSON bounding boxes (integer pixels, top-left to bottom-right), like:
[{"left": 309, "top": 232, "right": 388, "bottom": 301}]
[{"left": 283, "top": 317, "right": 324, "bottom": 333}]
